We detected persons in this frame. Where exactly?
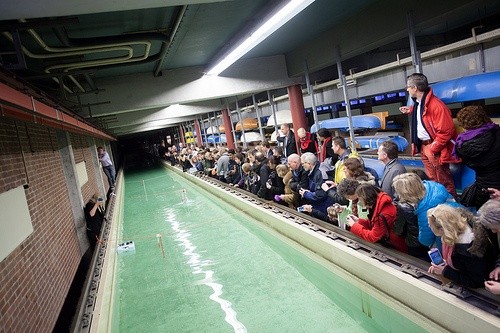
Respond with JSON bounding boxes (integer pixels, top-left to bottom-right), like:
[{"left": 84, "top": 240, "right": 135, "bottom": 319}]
[
  {"left": 275, "top": 136, "right": 500, "bottom": 296},
  {"left": 451, "top": 106, "right": 500, "bottom": 210},
  {"left": 317, "top": 128, "right": 338, "bottom": 165},
  {"left": 164, "top": 141, "right": 286, "bottom": 205},
  {"left": 276, "top": 124, "right": 297, "bottom": 157},
  {"left": 298, "top": 128, "right": 318, "bottom": 155},
  {"left": 84, "top": 193, "right": 107, "bottom": 244},
  {"left": 398, "top": 73, "right": 457, "bottom": 202},
  {"left": 97, "top": 147, "right": 116, "bottom": 187}
]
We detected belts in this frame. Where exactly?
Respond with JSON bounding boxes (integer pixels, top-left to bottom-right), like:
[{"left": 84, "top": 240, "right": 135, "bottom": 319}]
[{"left": 420, "top": 140, "right": 432, "bottom": 145}]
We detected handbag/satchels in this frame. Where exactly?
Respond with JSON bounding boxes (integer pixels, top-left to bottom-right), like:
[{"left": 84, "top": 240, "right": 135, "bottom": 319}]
[{"left": 378, "top": 214, "right": 395, "bottom": 248}]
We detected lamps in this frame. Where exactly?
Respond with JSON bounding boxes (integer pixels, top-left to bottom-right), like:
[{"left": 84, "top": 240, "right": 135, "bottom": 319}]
[{"left": 205, "top": 0, "right": 317, "bottom": 76}]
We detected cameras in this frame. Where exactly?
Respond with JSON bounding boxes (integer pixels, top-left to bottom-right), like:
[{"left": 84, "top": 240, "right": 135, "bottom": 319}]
[{"left": 97, "top": 197, "right": 104, "bottom": 203}]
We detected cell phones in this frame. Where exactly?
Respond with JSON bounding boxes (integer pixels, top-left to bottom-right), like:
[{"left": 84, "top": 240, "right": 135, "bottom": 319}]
[
  {"left": 298, "top": 207, "right": 305, "bottom": 212},
  {"left": 347, "top": 216, "right": 354, "bottom": 220},
  {"left": 325, "top": 183, "right": 332, "bottom": 187},
  {"left": 428, "top": 248, "right": 445, "bottom": 267}
]
